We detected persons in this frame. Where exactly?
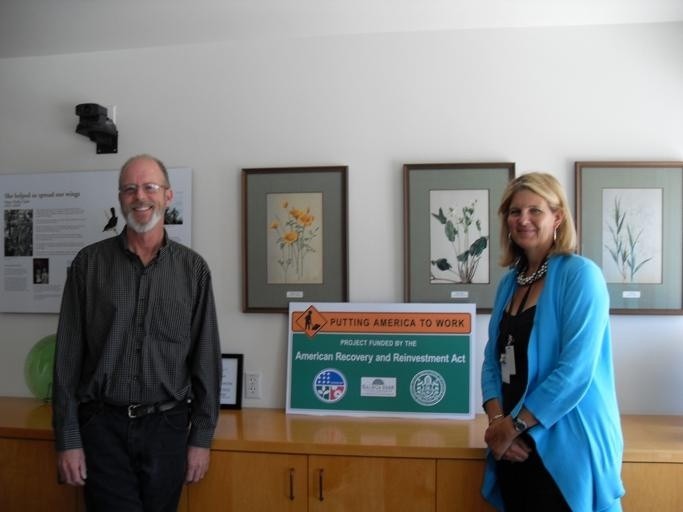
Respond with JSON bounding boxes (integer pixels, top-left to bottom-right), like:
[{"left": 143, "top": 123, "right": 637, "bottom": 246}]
[
  {"left": 475, "top": 174, "right": 627, "bottom": 512},
  {"left": 52, "top": 154, "right": 225, "bottom": 512}
]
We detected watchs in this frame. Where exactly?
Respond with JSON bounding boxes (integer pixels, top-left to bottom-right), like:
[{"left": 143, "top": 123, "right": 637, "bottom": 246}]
[{"left": 513, "top": 418, "right": 528, "bottom": 432}]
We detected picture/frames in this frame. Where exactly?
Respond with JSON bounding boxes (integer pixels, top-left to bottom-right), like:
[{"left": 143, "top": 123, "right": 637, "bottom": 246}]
[
  {"left": 573, "top": 158, "right": 683, "bottom": 317},
  {"left": 403, "top": 162, "right": 516, "bottom": 317},
  {"left": 220, "top": 353, "right": 244, "bottom": 408},
  {"left": 241, "top": 166, "right": 350, "bottom": 315}
]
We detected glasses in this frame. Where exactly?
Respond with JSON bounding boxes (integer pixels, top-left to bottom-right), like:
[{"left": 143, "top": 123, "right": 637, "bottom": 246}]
[{"left": 119, "top": 182, "right": 169, "bottom": 197}]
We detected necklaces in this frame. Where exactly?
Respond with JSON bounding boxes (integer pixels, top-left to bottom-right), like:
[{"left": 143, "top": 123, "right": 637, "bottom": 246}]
[{"left": 515, "top": 260, "right": 548, "bottom": 283}]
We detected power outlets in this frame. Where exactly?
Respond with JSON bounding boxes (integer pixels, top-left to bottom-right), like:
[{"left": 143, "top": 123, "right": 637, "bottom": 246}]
[{"left": 244, "top": 373, "right": 262, "bottom": 398}]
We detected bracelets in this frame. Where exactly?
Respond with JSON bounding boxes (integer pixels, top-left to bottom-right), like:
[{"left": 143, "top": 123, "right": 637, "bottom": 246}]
[{"left": 489, "top": 414, "right": 504, "bottom": 424}]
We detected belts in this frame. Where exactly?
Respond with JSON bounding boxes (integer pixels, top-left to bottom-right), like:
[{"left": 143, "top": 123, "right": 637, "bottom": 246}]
[{"left": 87, "top": 399, "right": 181, "bottom": 421}]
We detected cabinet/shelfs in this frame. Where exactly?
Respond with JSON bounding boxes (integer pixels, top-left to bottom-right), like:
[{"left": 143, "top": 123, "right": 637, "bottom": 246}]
[
  {"left": 438, "top": 413, "right": 683, "bottom": 510},
  {"left": 188, "top": 407, "right": 437, "bottom": 509},
  {"left": 2, "top": 398, "right": 189, "bottom": 511}
]
